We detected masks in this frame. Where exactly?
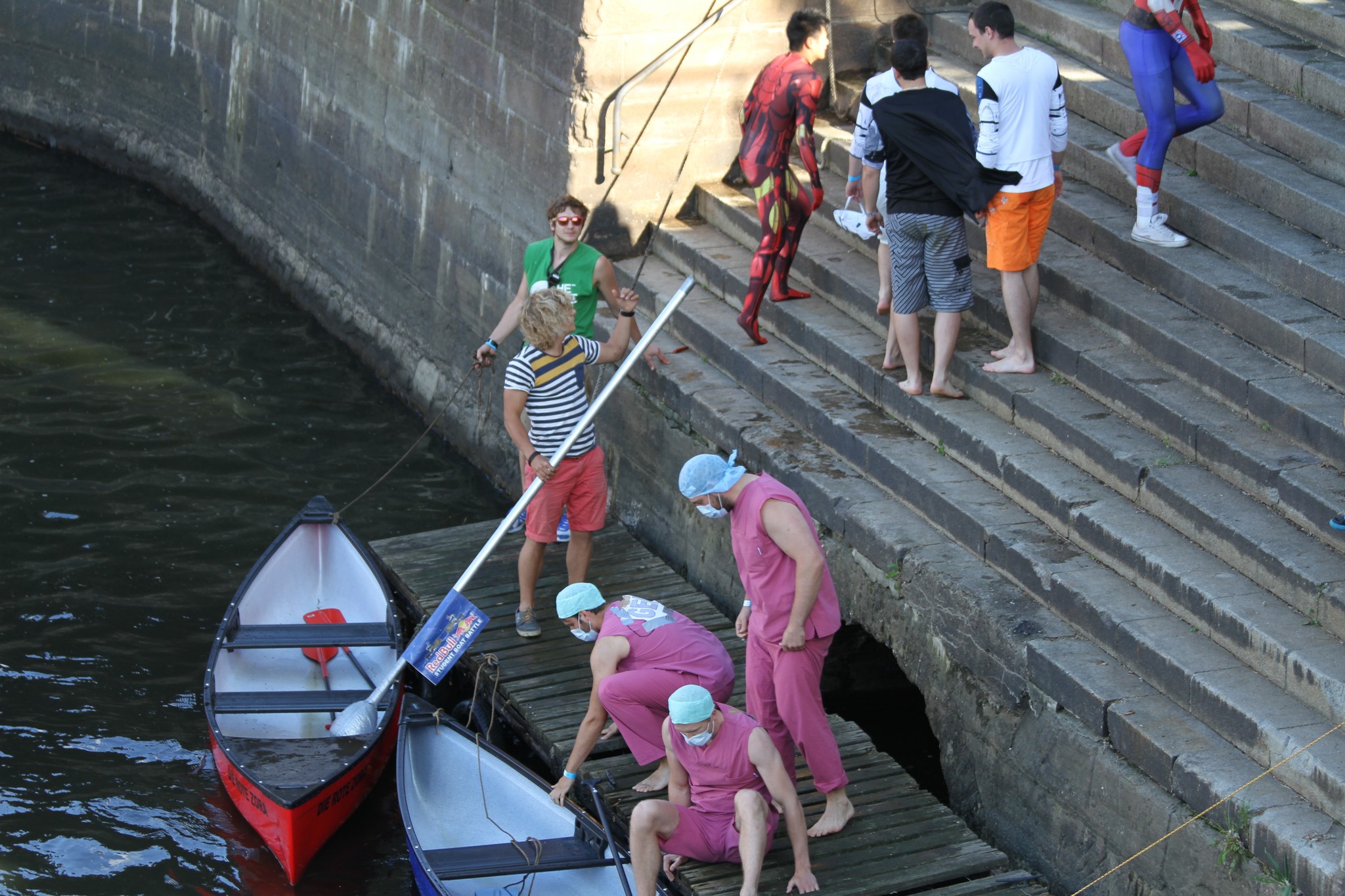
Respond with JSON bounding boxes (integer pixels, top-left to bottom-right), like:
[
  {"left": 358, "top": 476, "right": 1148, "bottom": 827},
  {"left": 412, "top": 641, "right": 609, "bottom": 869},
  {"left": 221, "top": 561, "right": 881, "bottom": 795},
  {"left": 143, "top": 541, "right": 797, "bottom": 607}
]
[
  {"left": 696, "top": 504, "right": 728, "bottom": 518},
  {"left": 679, "top": 730, "right": 714, "bottom": 747},
  {"left": 570, "top": 628, "right": 598, "bottom": 642}
]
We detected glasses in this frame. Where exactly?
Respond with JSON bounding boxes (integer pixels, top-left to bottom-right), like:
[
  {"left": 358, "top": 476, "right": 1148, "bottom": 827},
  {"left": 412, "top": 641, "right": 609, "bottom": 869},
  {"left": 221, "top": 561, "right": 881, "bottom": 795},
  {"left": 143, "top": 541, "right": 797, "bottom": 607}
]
[{"left": 552, "top": 216, "right": 584, "bottom": 226}]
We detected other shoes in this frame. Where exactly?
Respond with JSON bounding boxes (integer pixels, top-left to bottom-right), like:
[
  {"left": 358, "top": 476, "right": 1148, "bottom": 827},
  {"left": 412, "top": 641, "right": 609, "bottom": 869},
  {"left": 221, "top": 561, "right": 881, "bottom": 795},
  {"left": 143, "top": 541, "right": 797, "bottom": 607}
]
[{"left": 1329, "top": 515, "right": 1345, "bottom": 531}]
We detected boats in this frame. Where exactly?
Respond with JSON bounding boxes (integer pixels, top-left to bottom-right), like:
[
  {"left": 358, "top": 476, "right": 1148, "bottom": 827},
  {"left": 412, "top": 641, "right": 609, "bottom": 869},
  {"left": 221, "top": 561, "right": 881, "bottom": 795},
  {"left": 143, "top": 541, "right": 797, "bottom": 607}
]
[
  {"left": 397, "top": 692, "right": 673, "bottom": 896},
  {"left": 204, "top": 495, "right": 405, "bottom": 887}
]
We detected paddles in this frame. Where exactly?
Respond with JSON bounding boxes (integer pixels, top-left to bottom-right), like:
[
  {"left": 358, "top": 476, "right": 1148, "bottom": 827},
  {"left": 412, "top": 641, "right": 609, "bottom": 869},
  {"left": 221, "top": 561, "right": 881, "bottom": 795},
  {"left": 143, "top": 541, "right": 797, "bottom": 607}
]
[
  {"left": 580, "top": 777, "right": 633, "bottom": 896},
  {"left": 303, "top": 608, "right": 376, "bottom": 690},
  {"left": 301, "top": 647, "right": 338, "bottom": 730}
]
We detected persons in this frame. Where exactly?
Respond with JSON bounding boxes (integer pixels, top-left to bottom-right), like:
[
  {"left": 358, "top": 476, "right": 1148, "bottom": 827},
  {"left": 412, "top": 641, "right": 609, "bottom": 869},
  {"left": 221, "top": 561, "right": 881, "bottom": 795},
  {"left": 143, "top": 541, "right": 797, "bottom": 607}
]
[
  {"left": 846, "top": 3, "right": 1067, "bottom": 397},
  {"left": 678, "top": 450, "right": 853, "bottom": 836},
  {"left": 472, "top": 197, "right": 666, "bottom": 539},
  {"left": 735, "top": 8, "right": 822, "bottom": 345},
  {"left": 1106, "top": 0, "right": 1226, "bottom": 247},
  {"left": 503, "top": 290, "right": 638, "bottom": 641},
  {"left": 629, "top": 685, "right": 818, "bottom": 896},
  {"left": 549, "top": 583, "right": 735, "bottom": 807}
]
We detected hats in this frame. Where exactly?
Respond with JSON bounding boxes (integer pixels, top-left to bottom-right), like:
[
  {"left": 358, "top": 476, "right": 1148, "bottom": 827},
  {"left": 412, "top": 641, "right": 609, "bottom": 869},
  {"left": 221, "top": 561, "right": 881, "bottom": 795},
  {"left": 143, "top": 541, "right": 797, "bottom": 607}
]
[
  {"left": 668, "top": 684, "right": 714, "bottom": 724},
  {"left": 556, "top": 582, "right": 606, "bottom": 619}
]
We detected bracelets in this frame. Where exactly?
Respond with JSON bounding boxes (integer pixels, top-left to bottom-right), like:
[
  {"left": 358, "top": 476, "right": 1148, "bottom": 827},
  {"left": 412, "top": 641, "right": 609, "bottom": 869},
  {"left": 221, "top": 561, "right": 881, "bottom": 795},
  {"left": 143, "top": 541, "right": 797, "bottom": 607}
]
[
  {"left": 485, "top": 337, "right": 498, "bottom": 351},
  {"left": 619, "top": 310, "right": 636, "bottom": 317},
  {"left": 527, "top": 451, "right": 540, "bottom": 466},
  {"left": 848, "top": 174, "right": 861, "bottom": 182},
  {"left": 865, "top": 210, "right": 878, "bottom": 216},
  {"left": 1053, "top": 164, "right": 1062, "bottom": 170},
  {"left": 743, "top": 600, "right": 753, "bottom": 606},
  {"left": 563, "top": 769, "right": 576, "bottom": 780}
]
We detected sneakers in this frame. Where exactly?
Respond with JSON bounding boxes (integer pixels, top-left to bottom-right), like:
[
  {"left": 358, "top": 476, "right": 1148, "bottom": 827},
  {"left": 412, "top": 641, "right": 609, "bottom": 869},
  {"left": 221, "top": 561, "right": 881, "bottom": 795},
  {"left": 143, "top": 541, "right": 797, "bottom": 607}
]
[
  {"left": 1106, "top": 142, "right": 1137, "bottom": 188},
  {"left": 1131, "top": 213, "right": 1189, "bottom": 247},
  {"left": 556, "top": 513, "right": 570, "bottom": 543},
  {"left": 497, "top": 507, "right": 526, "bottom": 532},
  {"left": 515, "top": 606, "right": 541, "bottom": 637}
]
